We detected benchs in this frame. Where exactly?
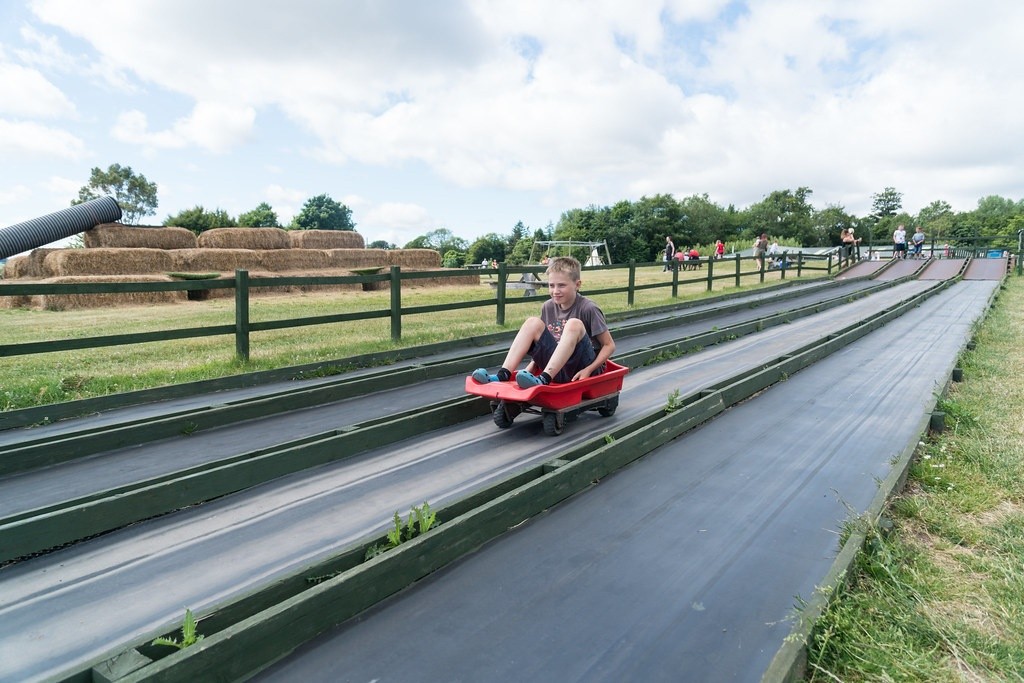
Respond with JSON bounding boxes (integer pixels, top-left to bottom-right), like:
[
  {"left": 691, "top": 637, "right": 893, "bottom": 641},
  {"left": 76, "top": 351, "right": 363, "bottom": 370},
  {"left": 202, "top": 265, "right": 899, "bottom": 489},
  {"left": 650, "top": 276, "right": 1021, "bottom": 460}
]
[{"left": 488, "top": 279, "right": 548, "bottom": 290}]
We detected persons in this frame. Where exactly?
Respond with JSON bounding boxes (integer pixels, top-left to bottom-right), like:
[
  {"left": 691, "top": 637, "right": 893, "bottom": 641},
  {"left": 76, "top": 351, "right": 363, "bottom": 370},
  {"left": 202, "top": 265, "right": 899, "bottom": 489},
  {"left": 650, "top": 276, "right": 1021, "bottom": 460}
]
[
  {"left": 482, "top": 258, "right": 498, "bottom": 269},
  {"left": 472, "top": 256, "right": 616, "bottom": 389},
  {"left": 663, "top": 236, "right": 724, "bottom": 272},
  {"left": 543, "top": 254, "right": 551, "bottom": 265},
  {"left": 841, "top": 223, "right": 925, "bottom": 267},
  {"left": 753, "top": 234, "right": 791, "bottom": 272}
]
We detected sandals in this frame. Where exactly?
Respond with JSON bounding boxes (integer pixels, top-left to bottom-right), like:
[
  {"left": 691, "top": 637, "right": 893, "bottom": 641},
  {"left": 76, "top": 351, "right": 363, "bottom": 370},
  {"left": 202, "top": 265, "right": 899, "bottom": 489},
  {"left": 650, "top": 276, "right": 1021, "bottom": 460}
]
[
  {"left": 471, "top": 367, "right": 499, "bottom": 384},
  {"left": 516, "top": 368, "right": 545, "bottom": 388}
]
